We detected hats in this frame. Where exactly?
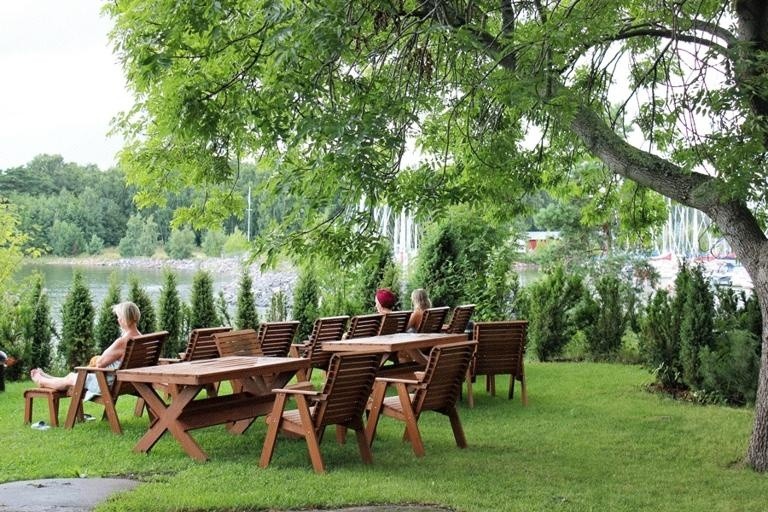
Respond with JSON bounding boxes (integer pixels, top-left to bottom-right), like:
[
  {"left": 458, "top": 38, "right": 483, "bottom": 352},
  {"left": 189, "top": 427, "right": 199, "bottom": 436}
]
[{"left": 376, "top": 288, "right": 397, "bottom": 308}]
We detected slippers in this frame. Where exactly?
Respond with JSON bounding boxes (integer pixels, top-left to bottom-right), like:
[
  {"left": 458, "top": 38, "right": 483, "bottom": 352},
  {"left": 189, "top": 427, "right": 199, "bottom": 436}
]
[
  {"left": 31, "top": 421, "right": 51, "bottom": 430},
  {"left": 84, "top": 414, "right": 96, "bottom": 421}
]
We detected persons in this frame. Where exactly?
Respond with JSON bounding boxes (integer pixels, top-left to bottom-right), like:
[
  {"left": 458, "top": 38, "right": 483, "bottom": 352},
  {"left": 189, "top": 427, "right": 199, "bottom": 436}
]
[
  {"left": 28, "top": 300, "right": 143, "bottom": 395},
  {"left": 405, "top": 288, "right": 433, "bottom": 333},
  {"left": 374, "top": 288, "right": 397, "bottom": 315}
]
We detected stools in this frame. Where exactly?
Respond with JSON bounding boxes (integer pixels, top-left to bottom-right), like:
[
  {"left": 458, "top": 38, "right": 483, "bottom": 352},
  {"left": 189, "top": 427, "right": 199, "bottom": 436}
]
[{"left": 24, "top": 383, "right": 89, "bottom": 427}]
